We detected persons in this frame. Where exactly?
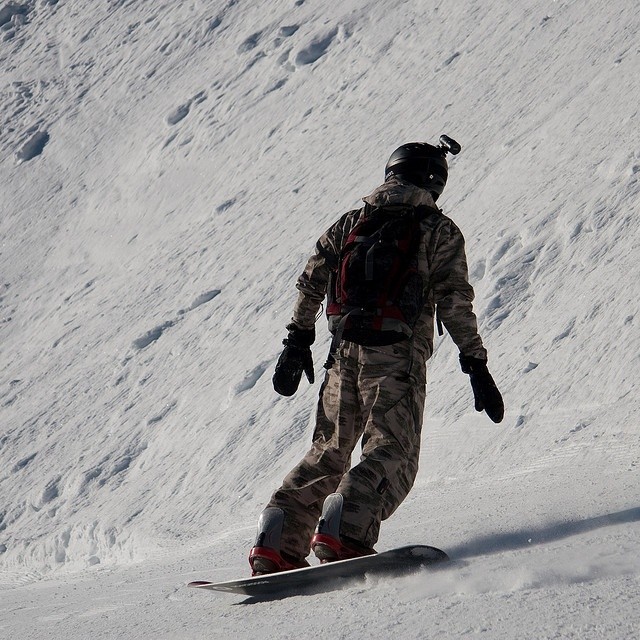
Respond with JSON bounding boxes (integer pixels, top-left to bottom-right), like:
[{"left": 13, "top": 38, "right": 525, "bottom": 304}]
[{"left": 250, "top": 143, "right": 502, "bottom": 577}]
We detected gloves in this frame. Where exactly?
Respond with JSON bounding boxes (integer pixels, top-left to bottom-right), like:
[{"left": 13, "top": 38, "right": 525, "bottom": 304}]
[
  {"left": 458, "top": 351, "right": 505, "bottom": 424},
  {"left": 272, "top": 339, "right": 315, "bottom": 396}
]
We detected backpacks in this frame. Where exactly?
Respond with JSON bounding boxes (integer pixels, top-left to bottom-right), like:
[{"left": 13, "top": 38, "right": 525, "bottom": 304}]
[{"left": 324, "top": 198, "right": 435, "bottom": 370}]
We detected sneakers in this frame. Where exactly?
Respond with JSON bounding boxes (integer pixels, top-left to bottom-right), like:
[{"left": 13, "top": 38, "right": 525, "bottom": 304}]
[
  {"left": 311, "top": 521, "right": 383, "bottom": 564},
  {"left": 248, "top": 531, "right": 311, "bottom": 577}
]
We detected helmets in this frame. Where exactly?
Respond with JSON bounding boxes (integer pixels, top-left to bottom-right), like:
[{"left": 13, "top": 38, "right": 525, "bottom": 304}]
[{"left": 385, "top": 141, "right": 450, "bottom": 202}]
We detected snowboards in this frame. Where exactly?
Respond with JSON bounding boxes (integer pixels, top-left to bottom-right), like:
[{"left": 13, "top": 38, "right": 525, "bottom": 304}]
[{"left": 188, "top": 546, "right": 448, "bottom": 597}]
